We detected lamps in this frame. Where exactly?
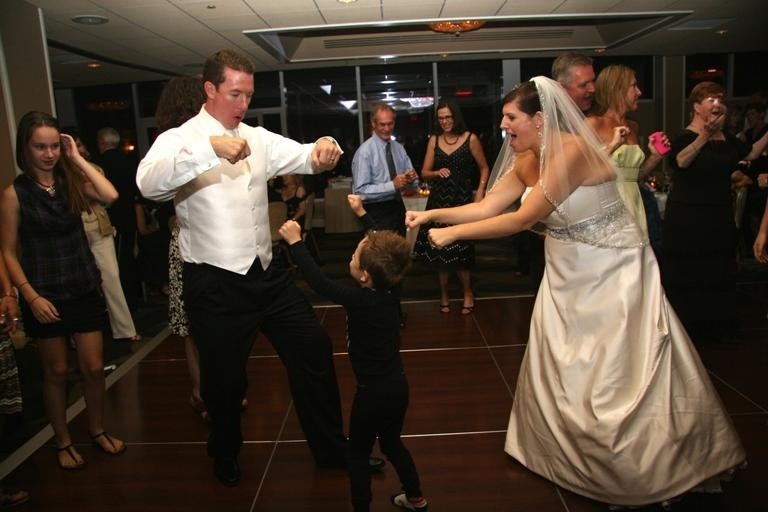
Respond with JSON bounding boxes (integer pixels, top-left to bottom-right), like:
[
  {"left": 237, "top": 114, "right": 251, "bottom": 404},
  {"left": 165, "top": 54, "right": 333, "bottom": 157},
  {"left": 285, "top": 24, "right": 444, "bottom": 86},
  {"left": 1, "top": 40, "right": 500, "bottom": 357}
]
[{"left": 429, "top": 20, "right": 486, "bottom": 36}]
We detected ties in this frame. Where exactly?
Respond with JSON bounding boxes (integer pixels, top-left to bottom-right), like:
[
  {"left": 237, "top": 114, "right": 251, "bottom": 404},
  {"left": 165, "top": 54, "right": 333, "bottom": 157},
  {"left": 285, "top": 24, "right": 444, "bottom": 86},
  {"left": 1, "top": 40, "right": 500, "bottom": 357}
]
[{"left": 385, "top": 142, "right": 397, "bottom": 181}]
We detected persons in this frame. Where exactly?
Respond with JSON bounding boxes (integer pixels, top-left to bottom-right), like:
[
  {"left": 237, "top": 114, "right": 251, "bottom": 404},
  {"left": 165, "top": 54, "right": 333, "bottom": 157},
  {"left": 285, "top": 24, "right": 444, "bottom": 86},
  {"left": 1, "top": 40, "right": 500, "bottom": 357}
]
[
  {"left": 403, "top": 76, "right": 747, "bottom": 510},
  {"left": 135, "top": 50, "right": 385, "bottom": 488},
  {"left": 552, "top": 52, "right": 766, "bottom": 342},
  {"left": 351, "top": 100, "right": 490, "bottom": 316},
  {"left": 278, "top": 195, "right": 427, "bottom": 511},
  {"left": 3, "top": 47, "right": 307, "bottom": 510}
]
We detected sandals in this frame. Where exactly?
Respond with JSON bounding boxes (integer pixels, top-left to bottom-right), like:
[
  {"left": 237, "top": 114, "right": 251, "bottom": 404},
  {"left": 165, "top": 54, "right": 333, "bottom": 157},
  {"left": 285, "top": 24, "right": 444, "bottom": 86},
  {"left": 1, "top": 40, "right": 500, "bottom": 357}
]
[
  {"left": 439, "top": 303, "right": 450, "bottom": 314},
  {"left": 86, "top": 428, "right": 127, "bottom": 456},
  {"left": 186, "top": 391, "right": 209, "bottom": 425},
  {"left": 54, "top": 440, "right": 88, "bottom": 471},
  {"left": 461, "top": 298, "right": 476, "bottom": 315},
  {"left": 0, "top": 488, "right": 31, "bottom": 512}
]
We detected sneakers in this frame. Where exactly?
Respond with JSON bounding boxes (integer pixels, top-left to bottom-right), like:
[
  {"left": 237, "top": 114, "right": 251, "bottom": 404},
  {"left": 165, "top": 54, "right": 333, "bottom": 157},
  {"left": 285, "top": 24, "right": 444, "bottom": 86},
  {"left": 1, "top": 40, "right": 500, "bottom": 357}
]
[{"left": 391, "top": 493, "right": 428, "bottom": 512}]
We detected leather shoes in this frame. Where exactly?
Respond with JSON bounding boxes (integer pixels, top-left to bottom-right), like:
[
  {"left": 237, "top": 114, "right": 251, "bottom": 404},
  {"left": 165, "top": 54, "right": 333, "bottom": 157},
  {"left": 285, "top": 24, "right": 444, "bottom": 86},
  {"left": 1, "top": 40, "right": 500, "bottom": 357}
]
[
  {"left": 368, "top": 456, "right": 385, "bottom": 472},
  {"left": 211, "top": 446, "right": 241, "bottom": 487}
]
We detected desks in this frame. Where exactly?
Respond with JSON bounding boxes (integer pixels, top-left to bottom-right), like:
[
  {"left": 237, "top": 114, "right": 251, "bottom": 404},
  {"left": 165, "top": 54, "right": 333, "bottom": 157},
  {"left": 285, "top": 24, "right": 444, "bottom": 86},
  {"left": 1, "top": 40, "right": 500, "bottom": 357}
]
[{"left": 324, "top": 187, "right": 364, "bottom": 234}]
